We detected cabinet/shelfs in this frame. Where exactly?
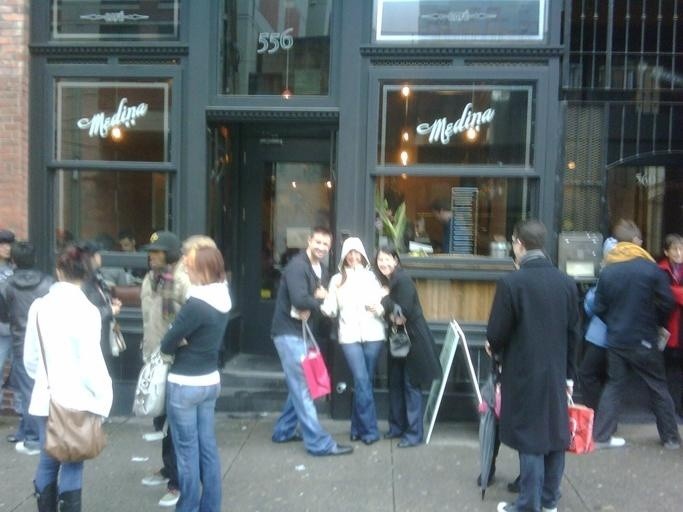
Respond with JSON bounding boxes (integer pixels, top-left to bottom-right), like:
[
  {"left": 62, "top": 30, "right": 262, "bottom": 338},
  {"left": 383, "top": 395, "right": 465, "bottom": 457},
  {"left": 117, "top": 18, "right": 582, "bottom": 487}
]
[{"left": 448, "top": 186, "right": 481, "bottom": 253}]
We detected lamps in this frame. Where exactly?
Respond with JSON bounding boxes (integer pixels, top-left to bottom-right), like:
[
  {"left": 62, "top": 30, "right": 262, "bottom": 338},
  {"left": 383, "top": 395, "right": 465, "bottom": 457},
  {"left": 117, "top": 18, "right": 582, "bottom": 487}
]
[{"left": 278, "top": 43, "right": 295, "bottom": 103}]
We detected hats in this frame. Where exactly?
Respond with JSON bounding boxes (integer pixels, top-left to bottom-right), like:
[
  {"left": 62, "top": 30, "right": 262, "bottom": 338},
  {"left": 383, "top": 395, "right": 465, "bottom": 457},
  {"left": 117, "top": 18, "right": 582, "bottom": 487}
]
[{"left": 142, "top": 230, "right": 182, "bottom": 253}]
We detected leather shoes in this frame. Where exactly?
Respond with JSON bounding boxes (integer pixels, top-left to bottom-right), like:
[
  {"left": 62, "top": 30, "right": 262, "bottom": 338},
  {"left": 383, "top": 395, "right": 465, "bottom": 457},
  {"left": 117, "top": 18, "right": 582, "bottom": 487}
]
[{"left": 328, "top": 443, "right": 353, "bottom": 456}]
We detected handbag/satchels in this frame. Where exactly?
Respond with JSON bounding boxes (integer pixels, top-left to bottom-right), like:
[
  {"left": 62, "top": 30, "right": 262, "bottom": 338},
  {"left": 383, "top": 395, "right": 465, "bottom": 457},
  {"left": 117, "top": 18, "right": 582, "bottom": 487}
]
[
  {"left": 388, "top": 314, "right": 412, "bottom": 358},
  {"left": 131, "top": 347, "right": 169, "bottom": 419},
  {"left": 564, "top": 402, "right": 596, "bottom": 457},
  {"left": 44, "top": 398, "right": 107, "bottom": 462},
  {"left": 300, "top": 318, "right": 332, "bottom": 400},
  {"left": 108, "top": 319, "right": 127, "bottom": 357}
]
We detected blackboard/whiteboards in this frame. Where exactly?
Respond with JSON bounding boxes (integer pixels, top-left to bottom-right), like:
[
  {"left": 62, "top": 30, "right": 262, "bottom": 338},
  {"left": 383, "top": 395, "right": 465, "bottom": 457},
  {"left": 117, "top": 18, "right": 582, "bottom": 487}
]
[{"left": 422, "top": 321, "right": 460, "bottom": 445}]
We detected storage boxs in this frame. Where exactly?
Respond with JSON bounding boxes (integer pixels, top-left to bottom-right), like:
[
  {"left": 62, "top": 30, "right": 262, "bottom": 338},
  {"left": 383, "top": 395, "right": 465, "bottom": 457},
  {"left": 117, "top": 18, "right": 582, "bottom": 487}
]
[{"left": 557, "top": 229, "right": 604, "bottom": 282}]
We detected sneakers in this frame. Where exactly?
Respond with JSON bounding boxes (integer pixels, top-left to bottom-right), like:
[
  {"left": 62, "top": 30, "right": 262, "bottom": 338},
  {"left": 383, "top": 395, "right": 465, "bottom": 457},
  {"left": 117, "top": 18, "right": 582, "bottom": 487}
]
[
  {"left": 595, "top": 435, "right": 625, "bottom": 449},
  {"left": 351, "top": 435, "right": 361, "bottom": 441},
  {"left": 143, "top": 468, "right": 170, "bottom": 486},
  {"left": 145, "top": 431, "right": 165, "bottom": 441},
  {"left": 15, "top": 441, "right": 42, "bottom": 456},
  {"left": 288, "top": 431, "right": 303, "bottom": 442},
  {"left": 7, "top": 435, "right": 19, "bottom": 442},
  {"left": 159, "top": 490, "right": 181, "bottom": 507},
  {"left": 365, "top": 439, "right": 374, "bottom": 445},
  {"left": 383, "top": 431, "right": 402, "bottom": 439},
  {"left": 397, "top": 441, "right": 410, "bottom": 448},
  {"left": 495, "top": 477, "right": 557, "bottom": 512},
  {"left": 477, "top": 467, "right": 497, "bottom": 488},
  {"left": 663, "top": 438, "right": 681, "bottom": 450}
]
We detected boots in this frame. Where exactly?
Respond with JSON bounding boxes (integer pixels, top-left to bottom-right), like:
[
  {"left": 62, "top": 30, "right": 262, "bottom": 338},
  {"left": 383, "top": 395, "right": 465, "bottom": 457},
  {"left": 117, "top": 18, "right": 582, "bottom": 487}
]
[
  {"left": 58, "top": 488, "right": 82, "bottom": 512},
  {"left": 32, "top": 479, "right": 57, "bottom": 512}
]
